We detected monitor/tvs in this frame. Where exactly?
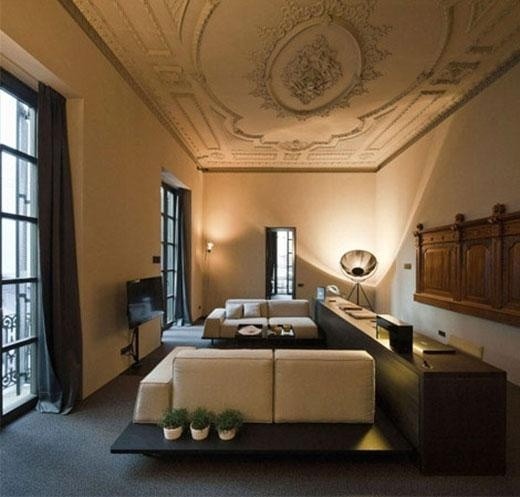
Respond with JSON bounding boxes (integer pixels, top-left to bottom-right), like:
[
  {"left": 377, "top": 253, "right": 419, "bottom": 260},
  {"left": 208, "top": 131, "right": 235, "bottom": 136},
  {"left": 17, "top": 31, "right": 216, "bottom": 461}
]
[{"left": 127, "top": 276, "right": 164, "bottom": 329}]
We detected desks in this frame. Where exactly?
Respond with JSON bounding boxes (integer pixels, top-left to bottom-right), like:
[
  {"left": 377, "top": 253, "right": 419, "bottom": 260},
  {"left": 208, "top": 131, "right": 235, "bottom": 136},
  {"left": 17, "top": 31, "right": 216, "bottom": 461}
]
[{"left": 319, "top": 298, "right": 507, "bottom": 474}]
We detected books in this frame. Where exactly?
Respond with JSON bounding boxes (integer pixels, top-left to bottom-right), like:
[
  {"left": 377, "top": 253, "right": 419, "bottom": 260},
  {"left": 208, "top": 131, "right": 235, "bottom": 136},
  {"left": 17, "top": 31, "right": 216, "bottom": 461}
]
[
  {"left": 346, "top": 310, "right": 377, "bottom": 319},
  {"left": 338, "top": 303, "right": 362, "bottom": 311}
]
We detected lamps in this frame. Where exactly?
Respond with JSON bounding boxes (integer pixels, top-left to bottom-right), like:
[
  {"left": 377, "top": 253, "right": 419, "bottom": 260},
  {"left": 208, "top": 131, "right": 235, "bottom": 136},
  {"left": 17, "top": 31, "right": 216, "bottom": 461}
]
[{"left": 340, "top": 249, "right": 378, "bottom": 313}]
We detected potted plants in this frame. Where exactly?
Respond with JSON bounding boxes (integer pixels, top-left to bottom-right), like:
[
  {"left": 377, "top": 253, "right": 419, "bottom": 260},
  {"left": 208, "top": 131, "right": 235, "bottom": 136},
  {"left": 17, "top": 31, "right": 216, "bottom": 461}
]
[
  {"left": 188, "top": 409, "right": 212, "bottom": 441},
  {"left": 210, "top": 410, "right": 243, "bottom": 441},
  {"left": 162, "top": 407, "right": 188, "bottom": 440}
]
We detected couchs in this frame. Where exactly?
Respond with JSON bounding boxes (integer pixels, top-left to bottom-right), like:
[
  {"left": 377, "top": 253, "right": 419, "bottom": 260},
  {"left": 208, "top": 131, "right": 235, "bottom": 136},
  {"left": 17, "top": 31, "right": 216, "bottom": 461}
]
[
  {"left": 132, "top": 346, "right": 375, "bottom": 423},
  {"left": 202, "top": 300, "right": 317, "bottom": 343}
]
